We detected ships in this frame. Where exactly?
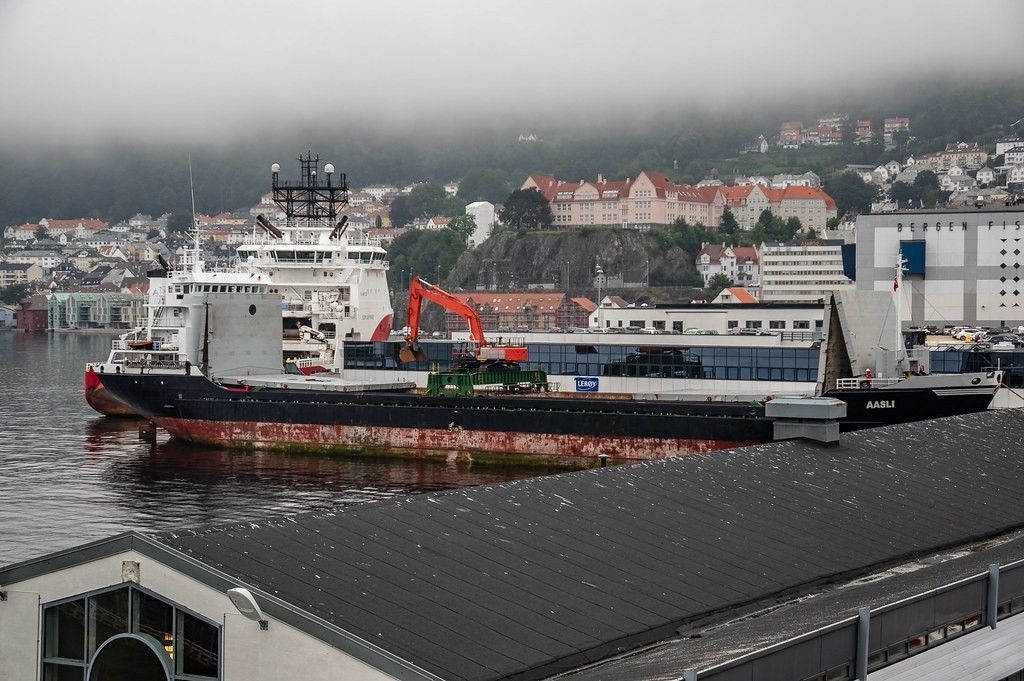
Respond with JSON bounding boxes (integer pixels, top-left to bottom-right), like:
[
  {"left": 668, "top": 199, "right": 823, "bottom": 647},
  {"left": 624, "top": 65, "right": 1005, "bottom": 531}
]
[{"left": 83, "top": 149, "right": 1006, "bottom": 470}]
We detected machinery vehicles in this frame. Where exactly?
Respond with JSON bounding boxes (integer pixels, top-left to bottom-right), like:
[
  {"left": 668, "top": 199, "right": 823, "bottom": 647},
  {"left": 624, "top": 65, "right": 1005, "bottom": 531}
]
[{"left": 399, "top": 276, "right": 550, "bottom": 397}]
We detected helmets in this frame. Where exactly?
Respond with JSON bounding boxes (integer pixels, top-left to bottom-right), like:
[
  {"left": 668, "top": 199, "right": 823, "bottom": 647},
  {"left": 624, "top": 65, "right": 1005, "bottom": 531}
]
[{"left": 866, "top": 368, "right": 870, "bottom": 372}]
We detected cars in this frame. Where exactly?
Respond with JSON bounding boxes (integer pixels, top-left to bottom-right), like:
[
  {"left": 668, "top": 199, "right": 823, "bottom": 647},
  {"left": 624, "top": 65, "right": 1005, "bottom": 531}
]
[{"left": 925, "top": 324, "right": 1024, "bottom": 344}]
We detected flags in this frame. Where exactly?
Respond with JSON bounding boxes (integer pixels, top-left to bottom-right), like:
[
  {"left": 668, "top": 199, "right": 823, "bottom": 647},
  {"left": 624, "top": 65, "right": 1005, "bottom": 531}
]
[{"left": 893, "top": 275, "right": 899, "bottom": 292}]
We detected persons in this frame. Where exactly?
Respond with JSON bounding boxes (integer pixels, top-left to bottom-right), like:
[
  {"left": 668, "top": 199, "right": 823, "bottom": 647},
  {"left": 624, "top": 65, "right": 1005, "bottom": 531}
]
[
  {"left": 919, "top": 365, "right": 930, "bottom": 376},
  {"left": 864, "top": 368, "right": 873, "bottom": 388}
]
